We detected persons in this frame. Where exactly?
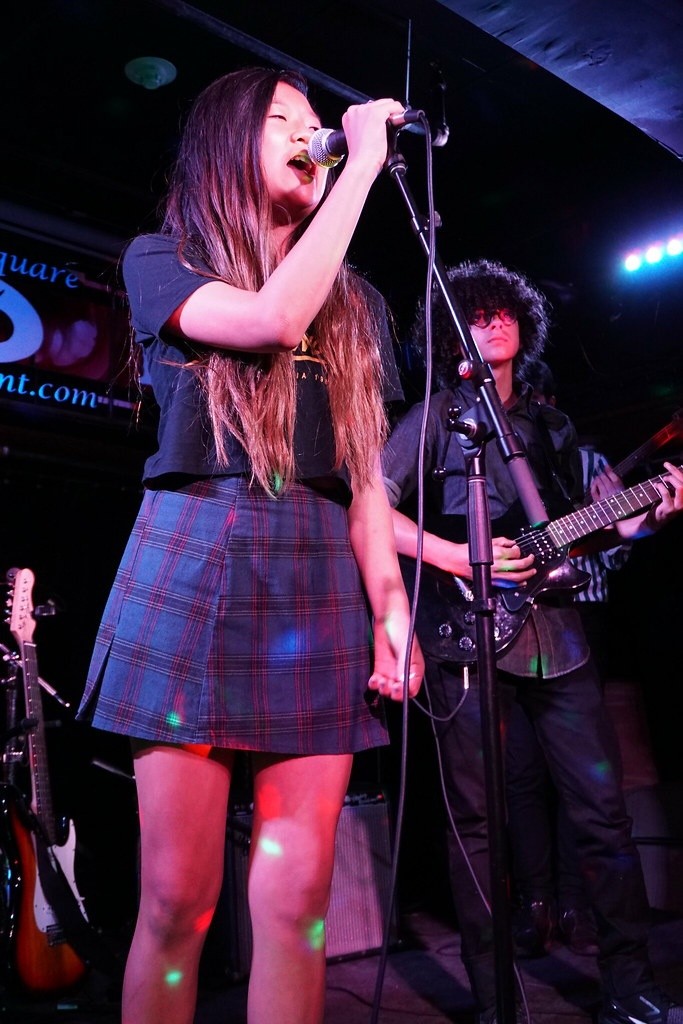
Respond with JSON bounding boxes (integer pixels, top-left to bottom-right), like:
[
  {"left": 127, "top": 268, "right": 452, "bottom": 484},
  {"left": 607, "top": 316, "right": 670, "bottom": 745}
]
[
  {"left": 76, "top": 67, "right": 425, "bottom": 1024},
  {"left": 378, "top": 259, "right": 683, "bottom": 1024}
]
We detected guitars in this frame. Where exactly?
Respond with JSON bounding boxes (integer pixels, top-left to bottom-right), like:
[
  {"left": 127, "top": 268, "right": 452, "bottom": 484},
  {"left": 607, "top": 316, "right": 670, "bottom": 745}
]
[
  {"left": 398, "top": 464, "right": 683, "bottom": 669},
  {"left": 2, "top": 562, "right": 98, "bottom": 1000},
  {"left": 573, "top": 409, "right": 683, "bottom": 514}
]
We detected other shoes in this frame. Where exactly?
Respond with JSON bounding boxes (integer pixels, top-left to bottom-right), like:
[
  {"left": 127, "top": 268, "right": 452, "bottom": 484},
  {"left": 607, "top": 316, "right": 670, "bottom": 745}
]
[
  {"left": 598, "top": 986, "right": 683, "bottom": 1024},
  {"left": 480, "top": 1007, "right": 536, "bottom": 1024}
]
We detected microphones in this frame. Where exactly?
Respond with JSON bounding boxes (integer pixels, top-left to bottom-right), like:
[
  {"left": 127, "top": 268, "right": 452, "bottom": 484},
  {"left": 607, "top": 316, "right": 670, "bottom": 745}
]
[{"left": 307, "top": 110, "right": 425, "bottom": 169}]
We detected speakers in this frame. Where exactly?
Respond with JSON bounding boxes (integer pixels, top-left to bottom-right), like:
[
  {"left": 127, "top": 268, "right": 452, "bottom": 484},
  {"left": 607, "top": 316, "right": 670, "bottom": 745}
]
[{"left": 220, "top": 792, "right": 405, "bottom": 982}]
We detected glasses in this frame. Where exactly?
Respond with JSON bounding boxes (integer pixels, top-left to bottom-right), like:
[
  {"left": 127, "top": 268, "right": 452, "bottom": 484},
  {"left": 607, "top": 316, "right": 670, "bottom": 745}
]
[{"left": 471, "top": 308, "right": 518, "bottom": 328}]
